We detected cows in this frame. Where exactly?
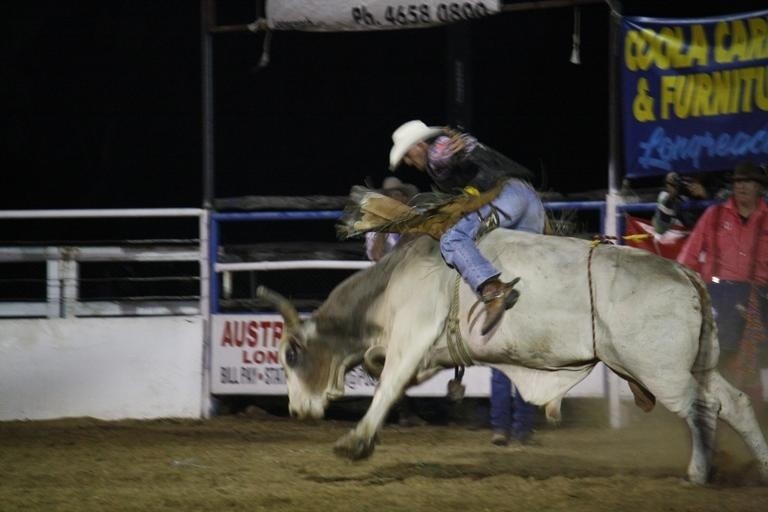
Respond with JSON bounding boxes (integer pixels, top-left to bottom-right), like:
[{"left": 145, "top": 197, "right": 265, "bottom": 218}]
[{"left": 257, "top": 228, "right": 768, "bottom": 486}]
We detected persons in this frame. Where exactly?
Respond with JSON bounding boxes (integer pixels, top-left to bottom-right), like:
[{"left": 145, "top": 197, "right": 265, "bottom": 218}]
[
  {"left": 676, "top": 158, "right": 768, "bottom": 403},
  {"left": 388, "top": 119, "right": 546, "bottom": 335},
  {"left": 677, "top": 164, "right": 706, "bottom": 199},
  {"left": 364, "top": 178, "right": 420, "bottom": 262},
  {"left": 652, "top": 172, "right": 690, "bottom": 233},
  {"left": 491, "top": 363, "right": 535, "bottom": 447}
]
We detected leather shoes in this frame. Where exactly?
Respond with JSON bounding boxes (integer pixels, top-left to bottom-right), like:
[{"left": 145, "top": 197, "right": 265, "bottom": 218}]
[
  {"left": 518, "top": 435, "right": 545, "bottom": 449},
  {"left": 492, "top": 433, "right": 508, "bottom": 446},
  {"left": 479, "top": 277, "right": 520, "bottom": 337}
]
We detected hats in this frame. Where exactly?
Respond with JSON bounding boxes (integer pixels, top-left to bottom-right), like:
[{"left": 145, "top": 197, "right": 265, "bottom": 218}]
[
  {"left": 723, "top": 159, "right": 767, "bottom": 181},
  {"left": 389, "top": 119, "right": 444, "bottom": 171},
  {"left": 665, "top": 171, "right": 682, "bottom": 187}
]
